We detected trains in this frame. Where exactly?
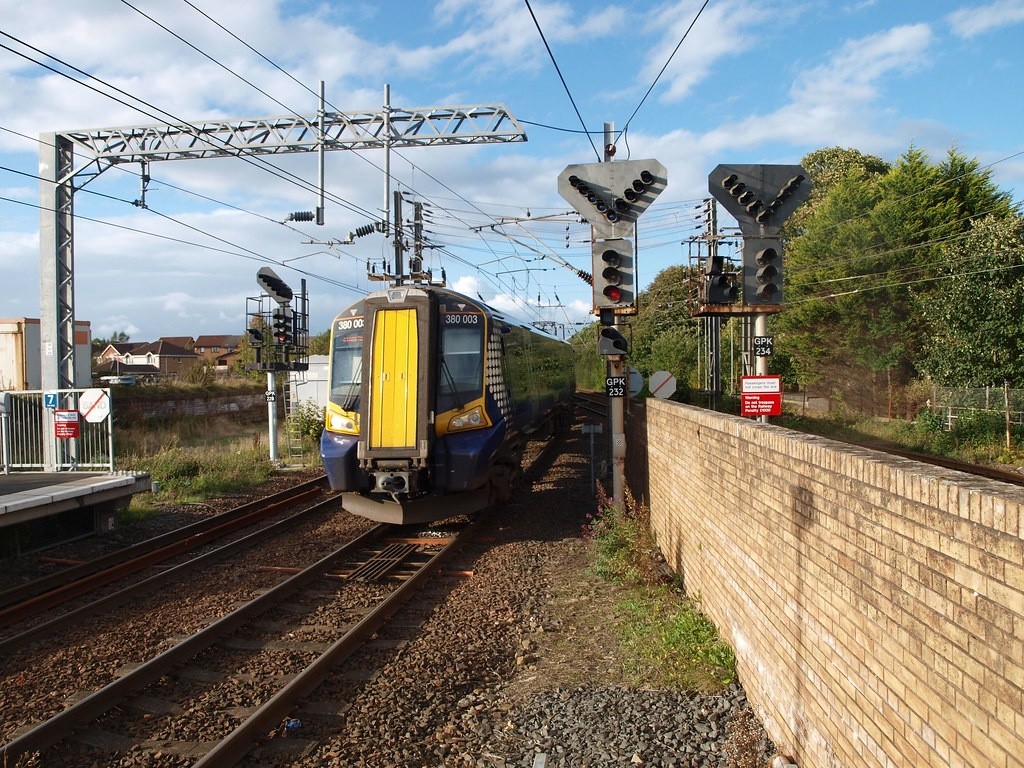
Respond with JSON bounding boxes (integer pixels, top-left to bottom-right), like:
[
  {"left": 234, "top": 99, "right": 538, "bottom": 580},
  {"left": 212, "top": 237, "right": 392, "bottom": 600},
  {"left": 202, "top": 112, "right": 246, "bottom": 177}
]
[{"left": 318, "top": 284, "right": 579, "bottom": 527}]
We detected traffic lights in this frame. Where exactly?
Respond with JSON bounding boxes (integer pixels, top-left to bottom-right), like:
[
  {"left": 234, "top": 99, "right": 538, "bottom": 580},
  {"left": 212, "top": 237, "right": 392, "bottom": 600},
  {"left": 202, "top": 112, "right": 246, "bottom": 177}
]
[
  {"left": 706, "top": 162, "right": 811, "bottom": 239},
  {"left": 706, "top": 276, "right": 738, "bottom": 305},
  {"left": 555, "top": 159, "right": 669, "bottom": 239},
  {"left": 272, "top": 307, "right": 293, "bottom": 349},
  {"left": 739, "top": 237, "right": 785, "bottom": 306},
  {"left": 590, "top": 240, "right": 635, "bottom": 310},
  {"left": 247, "top": 329, "right": 264, "bottom": 344},
  {"left": 598, "top": 307, "right": 616, "bottom": 324},
  {"left": 597, "top": 327, "right": 629, "bottom": 356},
  {"left": 704, "top": 256, "right": 725, "bottom": 276},
  {"left": 256, "top": 267, "right": 293, "bottom": 305}
]
